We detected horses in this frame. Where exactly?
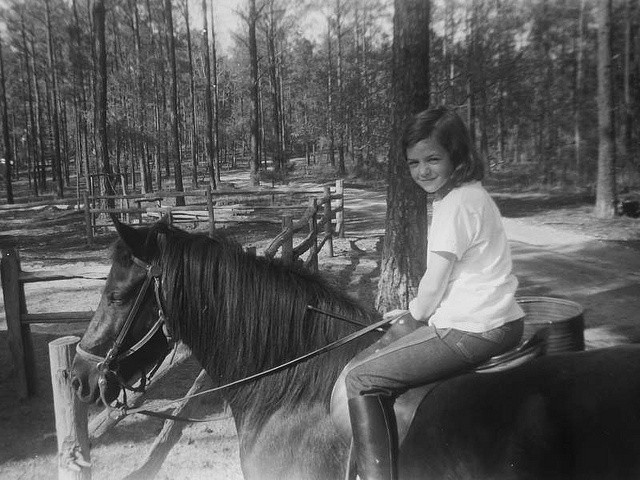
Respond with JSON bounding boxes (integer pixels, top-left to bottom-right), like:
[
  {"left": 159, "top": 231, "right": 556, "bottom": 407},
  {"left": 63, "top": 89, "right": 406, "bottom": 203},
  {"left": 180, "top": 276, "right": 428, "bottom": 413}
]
[{"left": 67, "top": 204, "right": 639, "bottom": 480}]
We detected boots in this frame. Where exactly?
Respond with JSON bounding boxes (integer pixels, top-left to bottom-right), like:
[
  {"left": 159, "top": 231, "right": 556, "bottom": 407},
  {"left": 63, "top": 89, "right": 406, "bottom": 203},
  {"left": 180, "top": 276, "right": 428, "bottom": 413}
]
[{"left": 348, "top": 395, "right": 398, "bottom": 479}]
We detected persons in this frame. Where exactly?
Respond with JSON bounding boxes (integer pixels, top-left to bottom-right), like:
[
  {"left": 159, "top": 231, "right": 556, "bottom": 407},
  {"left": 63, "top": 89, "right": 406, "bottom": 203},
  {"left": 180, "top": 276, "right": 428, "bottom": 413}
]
[{"left": 345, "top": 106, "right": 526, "bottom": 480}]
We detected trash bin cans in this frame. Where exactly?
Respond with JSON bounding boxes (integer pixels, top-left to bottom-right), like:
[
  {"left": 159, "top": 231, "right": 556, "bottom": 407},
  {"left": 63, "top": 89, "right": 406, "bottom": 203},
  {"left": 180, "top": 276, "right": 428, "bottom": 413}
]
[{"left": 516, "top": 296, "right": 584, "bottom": 354}]
